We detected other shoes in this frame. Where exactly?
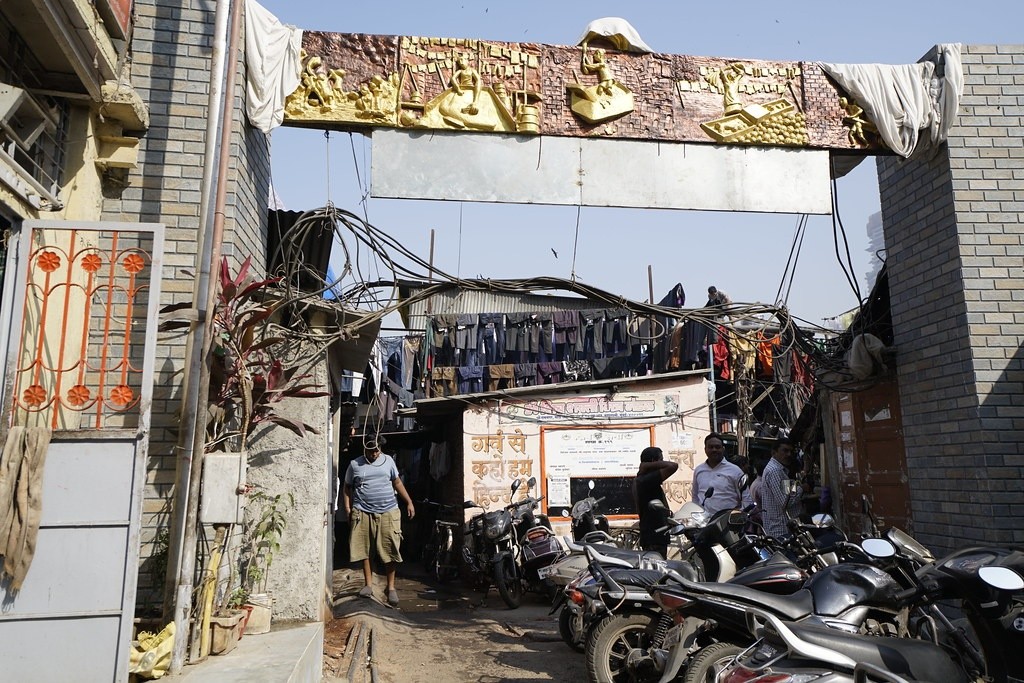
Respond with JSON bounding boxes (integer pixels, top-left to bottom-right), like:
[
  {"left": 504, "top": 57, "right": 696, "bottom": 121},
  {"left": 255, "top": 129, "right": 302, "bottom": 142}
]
[
  {"left": 359, "top": 585, "right": 373, "bottom": 597},
  {"left": 385, "top": 586, "right": 399, "bottom": 606}
]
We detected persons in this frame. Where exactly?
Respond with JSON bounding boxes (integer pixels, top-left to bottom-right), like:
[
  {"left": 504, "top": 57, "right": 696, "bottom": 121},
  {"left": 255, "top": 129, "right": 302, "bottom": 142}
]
[
  {"left": 692, "top": 432, "right": 757, "bottom": 517},
  {"left": 343, "top": 435, "right": 415, "bottom": 607},
  {"left": 705, "top": 286, "right": 732, "bottom": 308},
  {"left": 750, "top": 437, "right": 810, "bottom": 562},
  {"left": 632, "top": 446, "right": 678, "bottom": 561}
]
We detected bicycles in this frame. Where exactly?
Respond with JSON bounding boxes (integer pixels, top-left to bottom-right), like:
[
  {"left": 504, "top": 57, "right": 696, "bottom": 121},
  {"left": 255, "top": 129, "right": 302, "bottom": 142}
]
[{"left": 421, "top": 497, "right": 462, "bottom": 587}]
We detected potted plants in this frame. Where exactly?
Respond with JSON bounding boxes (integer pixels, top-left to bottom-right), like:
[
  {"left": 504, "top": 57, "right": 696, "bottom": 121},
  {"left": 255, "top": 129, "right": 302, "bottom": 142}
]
[
  {"left": 208, "top": 482, "right": 264, "bottom": 657},
  {"left": 244, "top": 493, "right": 297, "bottom": 635},
  {"left": 228, "top": 565, "right": 246, "bottom": 641},
  {"left": 237, "top": 566, "right": 264, "bottom": 639}
]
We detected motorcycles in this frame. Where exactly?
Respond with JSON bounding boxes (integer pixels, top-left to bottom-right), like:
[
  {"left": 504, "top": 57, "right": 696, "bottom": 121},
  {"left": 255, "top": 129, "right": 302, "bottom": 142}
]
[{"left": 461, "top": 476, "right": 1024, "bottom": 683}]
